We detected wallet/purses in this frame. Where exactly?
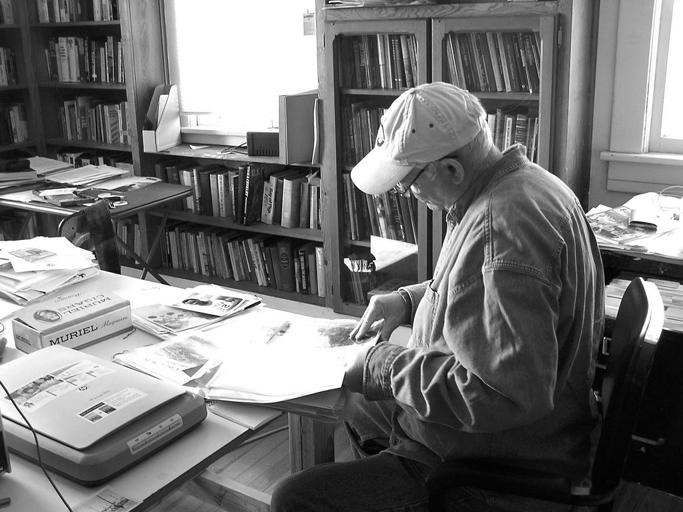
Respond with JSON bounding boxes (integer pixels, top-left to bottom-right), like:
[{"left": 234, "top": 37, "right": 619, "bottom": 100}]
[{"left": 630, "top": 209, "right": 657, "bottom": 230}]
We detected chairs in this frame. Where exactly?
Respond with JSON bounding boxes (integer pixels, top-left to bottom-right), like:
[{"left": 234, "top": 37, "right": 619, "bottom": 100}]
[
  {"left": 56, "top": 200, "right": 117, "bottom": 266},
  {"left": 443, "top": 275, "right": 663, "bottom": 511}
]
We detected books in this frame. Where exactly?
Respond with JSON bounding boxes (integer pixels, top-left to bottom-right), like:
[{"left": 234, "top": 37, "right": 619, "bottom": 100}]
[{"left": 605, "top": 271, "right": 683, "bottom": 334}]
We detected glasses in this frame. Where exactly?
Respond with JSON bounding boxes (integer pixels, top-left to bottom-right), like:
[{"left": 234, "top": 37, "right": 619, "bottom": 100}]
[{"left": 393, "top": 156, "right": 458, "bottom": 201}]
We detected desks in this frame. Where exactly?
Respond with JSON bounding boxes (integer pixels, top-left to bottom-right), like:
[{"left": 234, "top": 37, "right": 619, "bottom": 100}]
[
  {"left": 0, "top": 265, "right": 388, "bottom": 512},
  {"left": 0, "top": 174, "right": 193, "bottom": 284}
]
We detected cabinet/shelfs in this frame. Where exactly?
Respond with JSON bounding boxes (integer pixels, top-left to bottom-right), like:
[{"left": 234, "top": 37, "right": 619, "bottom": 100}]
[
  {"left": 26, "top": 0, "right": 165, "bottom": 280},
  {"left": 314, "top": 0, "right": 560, "bottom": 20},
  {"left": 0, "top": 1, "right": 49, "bottom": 241},
  {"left": 588, "top": 207, "right": 682, "bottom": 498},
  {"left": 147, "top": 143, "right": 328, "bottom": 305},
  {"left": 318, "top": 20, "right": 558, "bottom": 319}
]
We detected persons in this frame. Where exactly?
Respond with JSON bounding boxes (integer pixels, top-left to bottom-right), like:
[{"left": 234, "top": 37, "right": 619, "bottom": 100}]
[{"left": 271, "top": 81, "right": 606, "bottom": 512}]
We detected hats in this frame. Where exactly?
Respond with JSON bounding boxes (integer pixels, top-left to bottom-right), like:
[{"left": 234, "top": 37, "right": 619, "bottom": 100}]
[{"left": 350, "top": 82, "right": 487, "bottom": 195}]
